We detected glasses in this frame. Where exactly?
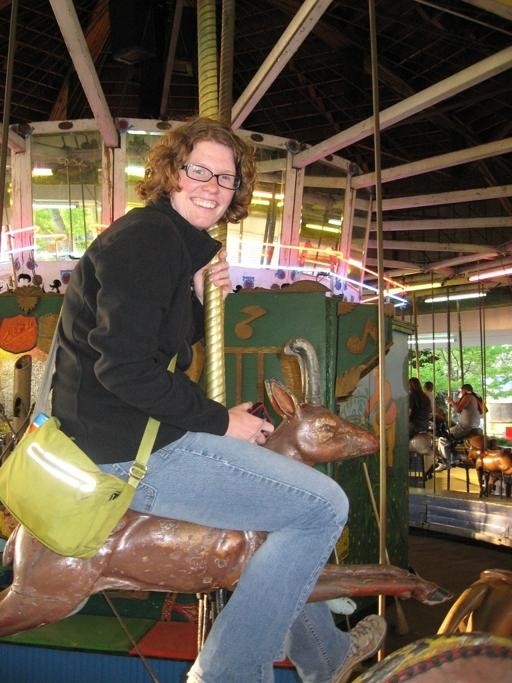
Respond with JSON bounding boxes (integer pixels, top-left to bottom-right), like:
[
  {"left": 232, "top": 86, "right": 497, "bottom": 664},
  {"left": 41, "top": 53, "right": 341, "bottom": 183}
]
[{"left": 181, "top": 164, "right": 242, "bottom": 190}]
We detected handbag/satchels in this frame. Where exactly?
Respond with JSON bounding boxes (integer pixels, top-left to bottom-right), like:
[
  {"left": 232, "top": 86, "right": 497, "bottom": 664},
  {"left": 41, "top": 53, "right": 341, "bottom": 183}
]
[{"left": 0, "top": 413, "right": 134, "bottom": 559}]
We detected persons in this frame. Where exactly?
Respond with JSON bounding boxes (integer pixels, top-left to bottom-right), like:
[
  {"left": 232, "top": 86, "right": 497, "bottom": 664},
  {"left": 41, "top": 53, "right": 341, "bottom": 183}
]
[
  {"left": 423, "top": 381, "right": 434, "bottom": 422},
  {"left": 435, "top": 384, "right": 488, "bottom": 472},
  {"left": 409, "top": 377, "right": 432, "bottom": 438},
  {"left": 49, "top": 118, "right": 388, "bottom": 682}
]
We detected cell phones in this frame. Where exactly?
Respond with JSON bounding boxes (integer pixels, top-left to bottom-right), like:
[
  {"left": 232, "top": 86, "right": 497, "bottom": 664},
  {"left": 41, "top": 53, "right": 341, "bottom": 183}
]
[{"left": 247, "top": 401, "right": 272, "bottom": 423}]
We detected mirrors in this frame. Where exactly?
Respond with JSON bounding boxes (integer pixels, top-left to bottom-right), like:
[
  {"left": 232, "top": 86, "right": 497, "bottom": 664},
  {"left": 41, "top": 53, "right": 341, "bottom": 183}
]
[
  {"left": 225, "top": 130, "right": 302, "bottom": 273},
  {"left": 344, "top": 165, "right": 377, "bottom": 295},
  {"left": 290, "top": 141, "right": 351, "bottom": 278},
  {"left": 24, "top": 119, "right": 112, "bottom": 296},
  {"left": 113, "top": 119, "right": 188, "bottom": 220}
]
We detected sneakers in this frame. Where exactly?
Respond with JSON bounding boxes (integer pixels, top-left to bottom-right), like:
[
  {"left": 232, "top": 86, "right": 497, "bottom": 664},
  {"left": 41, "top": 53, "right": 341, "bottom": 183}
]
[{"left": 332, "top": 614, "right": 387, "bottom": 683}]
